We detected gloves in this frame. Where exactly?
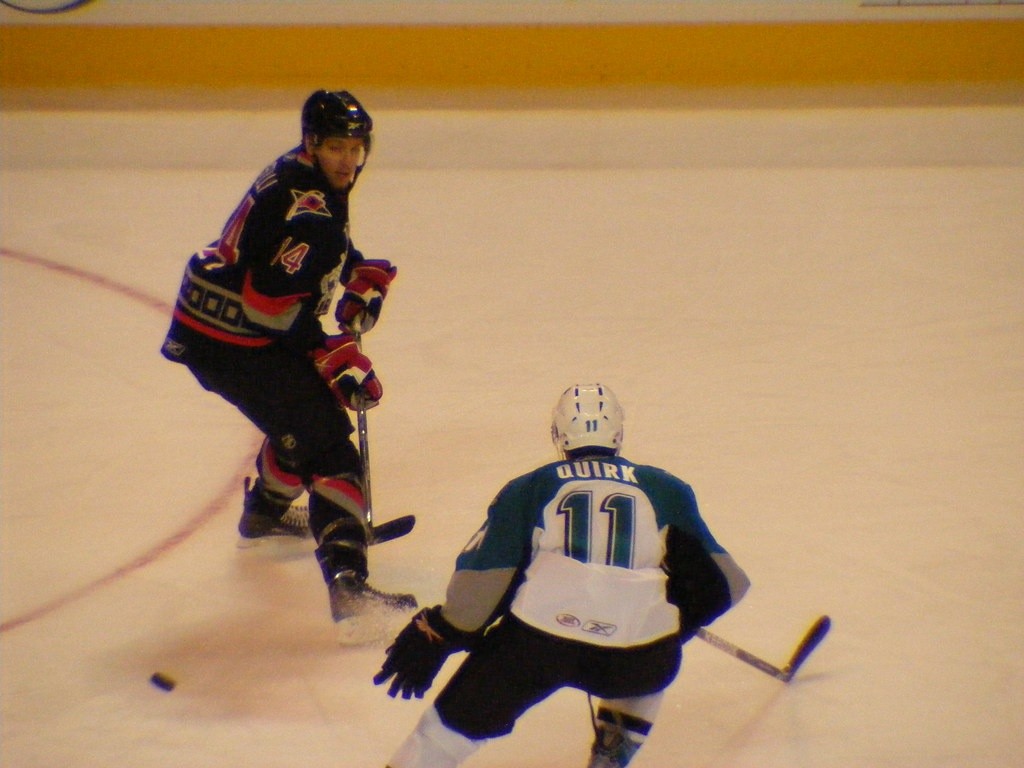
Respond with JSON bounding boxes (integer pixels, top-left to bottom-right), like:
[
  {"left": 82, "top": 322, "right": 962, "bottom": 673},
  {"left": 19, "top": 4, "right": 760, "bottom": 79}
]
[
  {"left": 307, "top": 335, "right": 383, "bottom": 411},
  {"left": 373, "top": 604, "right": 459, "bottom": 699},
  {"left": 334, "top": 259, "right": 396, "bottom": 335}
]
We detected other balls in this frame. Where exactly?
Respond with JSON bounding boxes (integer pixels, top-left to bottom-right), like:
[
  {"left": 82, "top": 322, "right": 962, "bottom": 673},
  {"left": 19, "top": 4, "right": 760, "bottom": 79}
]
[{"left": 149, "top": 671, "right": 176, "bottom": 692}]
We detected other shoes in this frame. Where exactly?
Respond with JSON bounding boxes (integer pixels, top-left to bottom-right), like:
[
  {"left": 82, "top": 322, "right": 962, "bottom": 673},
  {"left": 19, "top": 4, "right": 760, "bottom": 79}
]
[{"left": 588, "top": 725, "right": 629, "bottom": 768}]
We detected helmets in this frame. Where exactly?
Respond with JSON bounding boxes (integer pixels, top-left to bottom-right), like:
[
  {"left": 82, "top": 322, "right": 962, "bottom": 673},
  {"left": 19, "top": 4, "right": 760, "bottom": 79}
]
[
  {"left": 551, "top": 384, "right": 625, "bottom": 455},
  {"left": 301, "top": 89, "right": 374, "bottom": 138}
]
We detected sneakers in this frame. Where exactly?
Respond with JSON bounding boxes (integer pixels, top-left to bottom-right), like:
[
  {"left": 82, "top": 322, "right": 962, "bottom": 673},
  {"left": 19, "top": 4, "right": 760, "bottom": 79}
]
[
  {"left": 314, "top": 543, "right": 420, "bottom": 645},
  {"left": 235, "top": 476, "right": 316, "bottom": 548}
]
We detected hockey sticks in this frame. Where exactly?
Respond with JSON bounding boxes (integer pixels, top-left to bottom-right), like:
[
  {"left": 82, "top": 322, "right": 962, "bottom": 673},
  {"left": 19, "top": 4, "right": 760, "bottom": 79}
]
[
  {"left": 350, "top": 313, "right": 416, "bottom": 547},
  {"left": 696, "top": 611, "right": 833, "bottom": 684}
]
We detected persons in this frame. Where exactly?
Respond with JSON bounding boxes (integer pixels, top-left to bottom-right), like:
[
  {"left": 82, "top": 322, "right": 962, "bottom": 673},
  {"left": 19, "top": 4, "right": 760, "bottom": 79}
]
[
  {"left": 161, "top": 89, "right": 419, "bottom": 623},
  {"left": 374, "top": 383, "right": 751, "bottom": 768}
]
